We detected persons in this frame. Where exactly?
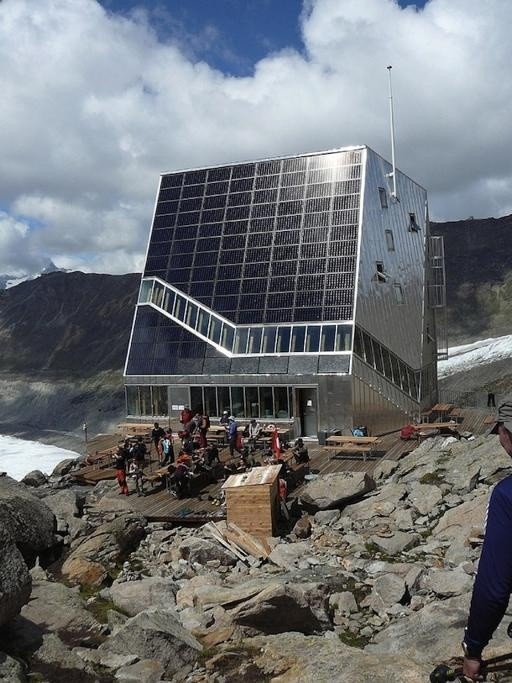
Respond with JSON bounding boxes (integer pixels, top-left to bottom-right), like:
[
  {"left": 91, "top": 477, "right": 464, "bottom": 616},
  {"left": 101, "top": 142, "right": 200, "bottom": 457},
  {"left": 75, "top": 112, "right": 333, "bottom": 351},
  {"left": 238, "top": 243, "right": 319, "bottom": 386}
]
[
  {"left": 460, "top": 389, "right": 512, "bottom": 681},
  {"left": 110, "top": 402, "right": 309, "bottom": 506},
  {"left": 484, "top": 377, "right": 498, "bottom": 406},
  {"left": 353, "top": 426, "right": 365, "bottom": 446}
]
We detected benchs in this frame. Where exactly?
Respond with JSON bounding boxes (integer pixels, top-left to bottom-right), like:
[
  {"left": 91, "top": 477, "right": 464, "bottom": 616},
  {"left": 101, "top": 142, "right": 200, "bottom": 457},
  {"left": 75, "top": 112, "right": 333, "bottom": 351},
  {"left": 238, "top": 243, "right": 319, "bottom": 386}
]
[
  {"left": 325, "top": 403, "right": 497, "bottom": 462},
  {"left": 77, "top": 426, "right": 291, "bottom": 493}
]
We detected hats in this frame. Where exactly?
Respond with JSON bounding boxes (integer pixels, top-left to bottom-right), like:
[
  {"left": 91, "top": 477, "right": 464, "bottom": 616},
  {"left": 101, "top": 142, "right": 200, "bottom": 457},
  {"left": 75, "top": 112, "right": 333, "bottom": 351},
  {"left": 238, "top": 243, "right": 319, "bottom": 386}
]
[
  {"left": 228, "top": 416, "right": 235, "bottom": 421},
  {"left": 485, "top": 395, "right": 512, "bottom": 437}
]
